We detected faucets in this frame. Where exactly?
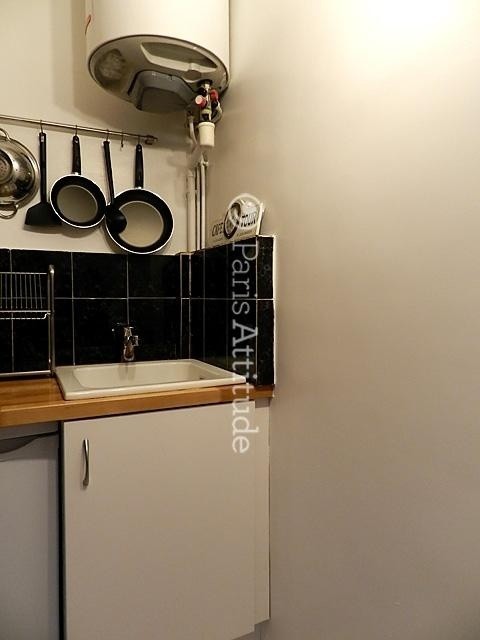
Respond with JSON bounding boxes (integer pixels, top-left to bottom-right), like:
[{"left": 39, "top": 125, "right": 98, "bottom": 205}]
[{"left": 120, "top": 322, "right": 138, "bottom": 362}]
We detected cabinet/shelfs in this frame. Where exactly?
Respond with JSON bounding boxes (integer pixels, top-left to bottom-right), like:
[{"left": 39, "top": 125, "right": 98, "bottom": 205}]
[{"left": 58, "top": 399, "right": 270, "bottom": 640}]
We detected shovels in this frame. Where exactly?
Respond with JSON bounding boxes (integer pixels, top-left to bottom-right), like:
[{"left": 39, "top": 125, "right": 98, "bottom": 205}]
[{"left": 24, "top": 132, "right": 63, "bottom": 226}]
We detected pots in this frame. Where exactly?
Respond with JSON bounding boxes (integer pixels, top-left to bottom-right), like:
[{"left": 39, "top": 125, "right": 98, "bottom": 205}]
[
  {"left": 0, "top": 127, "right": 39, "bottom": 220},
  {"left": 50, "top": 136, "right": 174, "bottom": 254}
]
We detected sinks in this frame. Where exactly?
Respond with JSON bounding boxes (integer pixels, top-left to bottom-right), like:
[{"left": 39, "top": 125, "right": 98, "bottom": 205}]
[{"left": 54, "top": 359, "right": 249, "bottom": 401}]
